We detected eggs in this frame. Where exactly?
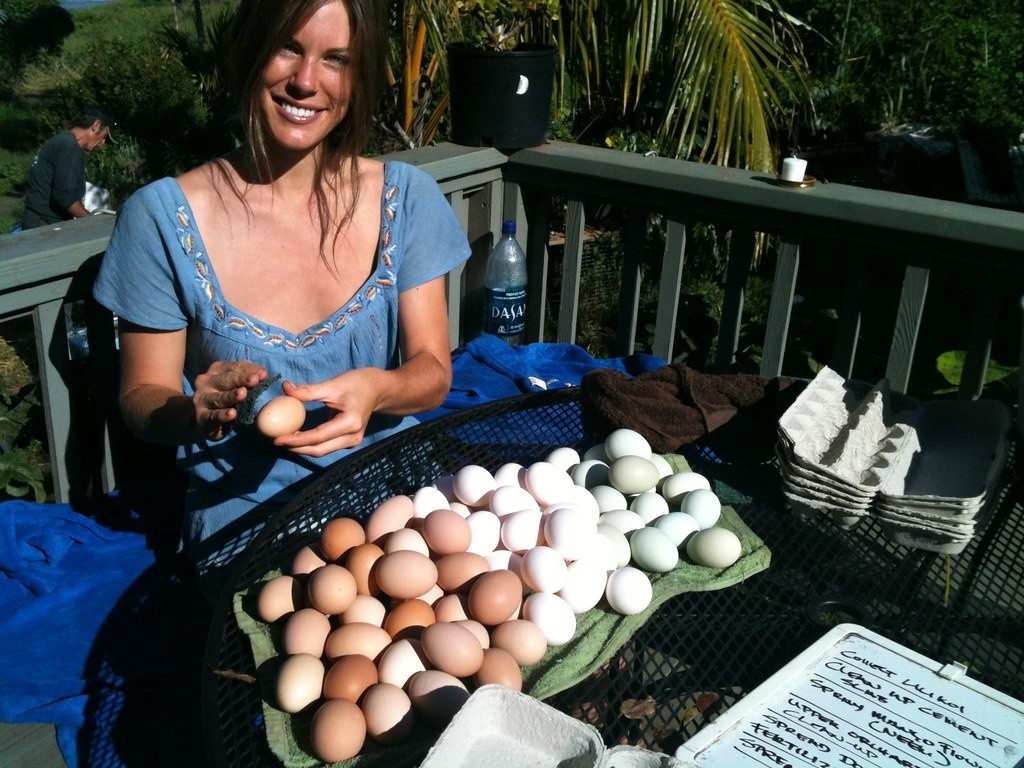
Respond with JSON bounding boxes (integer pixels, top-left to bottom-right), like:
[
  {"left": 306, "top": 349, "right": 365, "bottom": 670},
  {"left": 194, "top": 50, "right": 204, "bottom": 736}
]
[
  {"left": 259, "top": 428, "right": 742, "bottom": 759},
  {"left": 256, "top": 396, "right": 306, "bottom": 436}
]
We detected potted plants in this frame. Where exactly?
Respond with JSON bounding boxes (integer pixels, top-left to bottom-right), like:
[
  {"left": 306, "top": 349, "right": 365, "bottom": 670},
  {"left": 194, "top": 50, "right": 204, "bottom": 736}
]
[{"left": 446, "top": 0, "right": 561, "bottom": 147}]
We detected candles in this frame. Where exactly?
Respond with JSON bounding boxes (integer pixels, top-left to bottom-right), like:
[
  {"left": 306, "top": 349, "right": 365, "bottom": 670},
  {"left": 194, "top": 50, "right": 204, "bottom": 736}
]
[{"left": 781, "top": 155, "right": 807, "bottom": 182}]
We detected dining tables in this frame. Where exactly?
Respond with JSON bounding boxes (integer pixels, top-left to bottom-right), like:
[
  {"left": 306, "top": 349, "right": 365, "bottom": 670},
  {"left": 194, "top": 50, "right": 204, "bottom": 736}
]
[{"left": 195, "top": 370, "right": 1024, "bottom": 768}]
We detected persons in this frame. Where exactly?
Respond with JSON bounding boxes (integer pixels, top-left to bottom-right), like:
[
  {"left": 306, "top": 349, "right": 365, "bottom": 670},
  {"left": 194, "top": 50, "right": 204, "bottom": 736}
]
[
  {"left": 22, "top": 113, "right": 109, "bottom": 230},
  {"left": 94, "top": 0, "right": 474, "bottom": 607}
]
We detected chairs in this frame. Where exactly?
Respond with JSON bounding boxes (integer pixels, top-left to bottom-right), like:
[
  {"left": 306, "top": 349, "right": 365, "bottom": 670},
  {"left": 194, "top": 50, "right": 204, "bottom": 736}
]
[{"left": 87, "top": 261, "right": 474, "bottom": 768}]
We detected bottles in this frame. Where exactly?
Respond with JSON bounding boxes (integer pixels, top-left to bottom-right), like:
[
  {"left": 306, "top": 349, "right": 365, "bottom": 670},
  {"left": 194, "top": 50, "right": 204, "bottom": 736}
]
[{"left": 480, "top": 220, "right": 529, "bottom": 345}]
